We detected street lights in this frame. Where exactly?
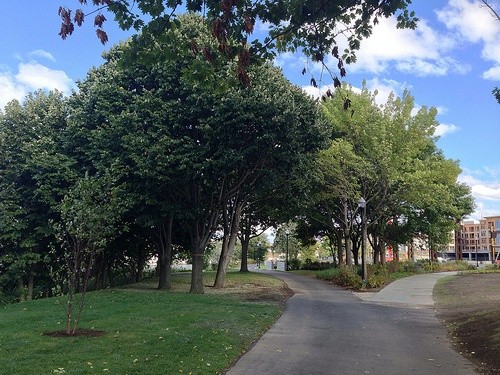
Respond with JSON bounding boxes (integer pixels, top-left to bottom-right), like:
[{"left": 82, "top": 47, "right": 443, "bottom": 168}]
[
  {"left": 286, "top": 230, "right": 290, "bottom": 271},
  {"left": 358, "top": 197, "right": 367, "bottom": 281}
]
